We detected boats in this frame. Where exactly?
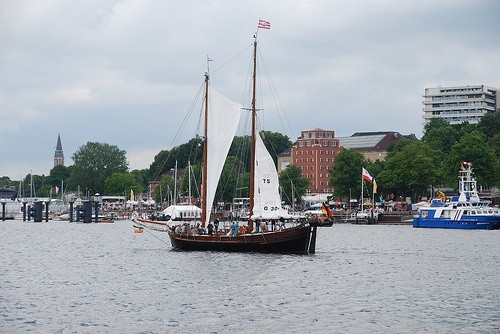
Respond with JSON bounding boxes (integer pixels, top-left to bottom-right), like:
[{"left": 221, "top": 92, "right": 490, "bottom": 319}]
[
  {"left": 141, "top": 158, "right": 382, "bottom": 226},
  {"left": 413, "top": 162, "right": 500, "bottom": 230}
]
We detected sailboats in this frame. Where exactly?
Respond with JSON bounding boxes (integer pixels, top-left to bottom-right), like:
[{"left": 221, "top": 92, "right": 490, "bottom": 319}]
[{"left": 130, "top": 18, "right": 317, "bottom": 256}]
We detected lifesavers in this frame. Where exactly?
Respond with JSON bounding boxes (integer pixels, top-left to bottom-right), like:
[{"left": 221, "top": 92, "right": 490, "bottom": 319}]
[
  {"left": 275, "top": 224, "right": 281, "bottom": 233},
  {"left": 131, "top": 212, "right": 138, "bottom": 220}
]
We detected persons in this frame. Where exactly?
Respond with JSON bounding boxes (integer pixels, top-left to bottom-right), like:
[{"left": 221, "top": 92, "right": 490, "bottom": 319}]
[
  {"left": 171, "top": 217, "right": 286, "bottom": 236},
  {"left": 305, "top": 214, "right": 330, "bottom": 222}
]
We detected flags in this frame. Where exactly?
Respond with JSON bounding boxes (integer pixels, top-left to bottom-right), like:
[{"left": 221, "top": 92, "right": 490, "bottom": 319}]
[
  {"left": 363, "top": 169, "right": 372, "bottom": 183},
  {"left": 258, "top": 20, "right": 270, "bottom": 29},
  {"left": 322, "top": 204, "right": 332, "bottom": 216},
  {"left": 374, "top": 181, "right": 377, "bottom": 193}
]
